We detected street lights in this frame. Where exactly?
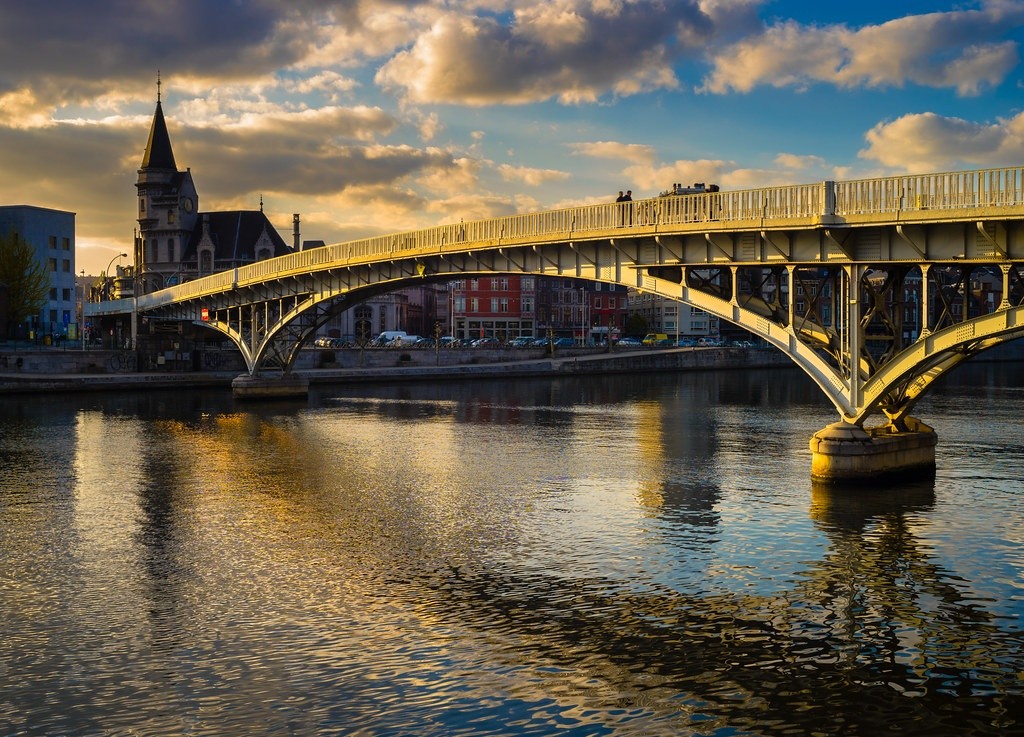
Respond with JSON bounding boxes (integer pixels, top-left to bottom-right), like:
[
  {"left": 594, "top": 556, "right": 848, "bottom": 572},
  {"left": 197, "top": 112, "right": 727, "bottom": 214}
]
[{"left": 107, "top": 253, "right": 127, "bottom": 301}]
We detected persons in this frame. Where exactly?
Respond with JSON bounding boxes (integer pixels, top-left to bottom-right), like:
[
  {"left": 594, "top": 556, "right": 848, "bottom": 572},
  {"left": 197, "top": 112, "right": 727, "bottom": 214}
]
[{"left": 616, "top": 190, "right": 632, "bottom": 228}]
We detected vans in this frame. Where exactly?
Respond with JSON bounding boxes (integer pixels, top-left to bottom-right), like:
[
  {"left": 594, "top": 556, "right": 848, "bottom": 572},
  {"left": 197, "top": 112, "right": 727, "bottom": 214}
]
[
  {"left": 378, "top": 332, "right": 407, "bottom": 340},
  {"left": 400, "top": 335, "right": 425, "bottom": 346}
]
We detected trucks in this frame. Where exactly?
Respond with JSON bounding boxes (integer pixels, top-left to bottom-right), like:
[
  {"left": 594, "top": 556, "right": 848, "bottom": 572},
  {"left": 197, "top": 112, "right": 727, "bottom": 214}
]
[{"left": 643, "top": 334, "right": 667, "bottom": 345}]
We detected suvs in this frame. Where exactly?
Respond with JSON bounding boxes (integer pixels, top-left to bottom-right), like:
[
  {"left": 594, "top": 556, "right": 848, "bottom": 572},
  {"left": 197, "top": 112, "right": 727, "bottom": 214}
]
[{"left": 698, "top": 338, "right": 719, "bottom": 347}]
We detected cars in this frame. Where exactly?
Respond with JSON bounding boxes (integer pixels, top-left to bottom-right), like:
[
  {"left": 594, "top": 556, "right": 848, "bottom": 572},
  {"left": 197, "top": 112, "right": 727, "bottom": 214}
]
[
  {"left": 472, "top": 338, "right": 490, "bottom": 347},
  {"left": 719, "top": 341, "right": 756, "bottom": 348},
  {"left": 546, "top": 338, "right": 573, "bottom": 348},
  {"left": 617, "top": 338, "right": 641, "bottom": 346},
  {"left": 508, "top": 336, "right": 550, "bottom": 347},
  {"left": 481, "top": 339, "right": 501, "bottom": 348},
  {"left": 654, "top": 339, "right": 673, "bottom": 346},
  {"left": 416, "top": 337, "right": 474, "bottom": 349},
  {"left": 679, "top": 340, "right": 695, "bottom": 346},
  {"left": 315, "top": 337, "right": 353, "bottom": 348}
]
